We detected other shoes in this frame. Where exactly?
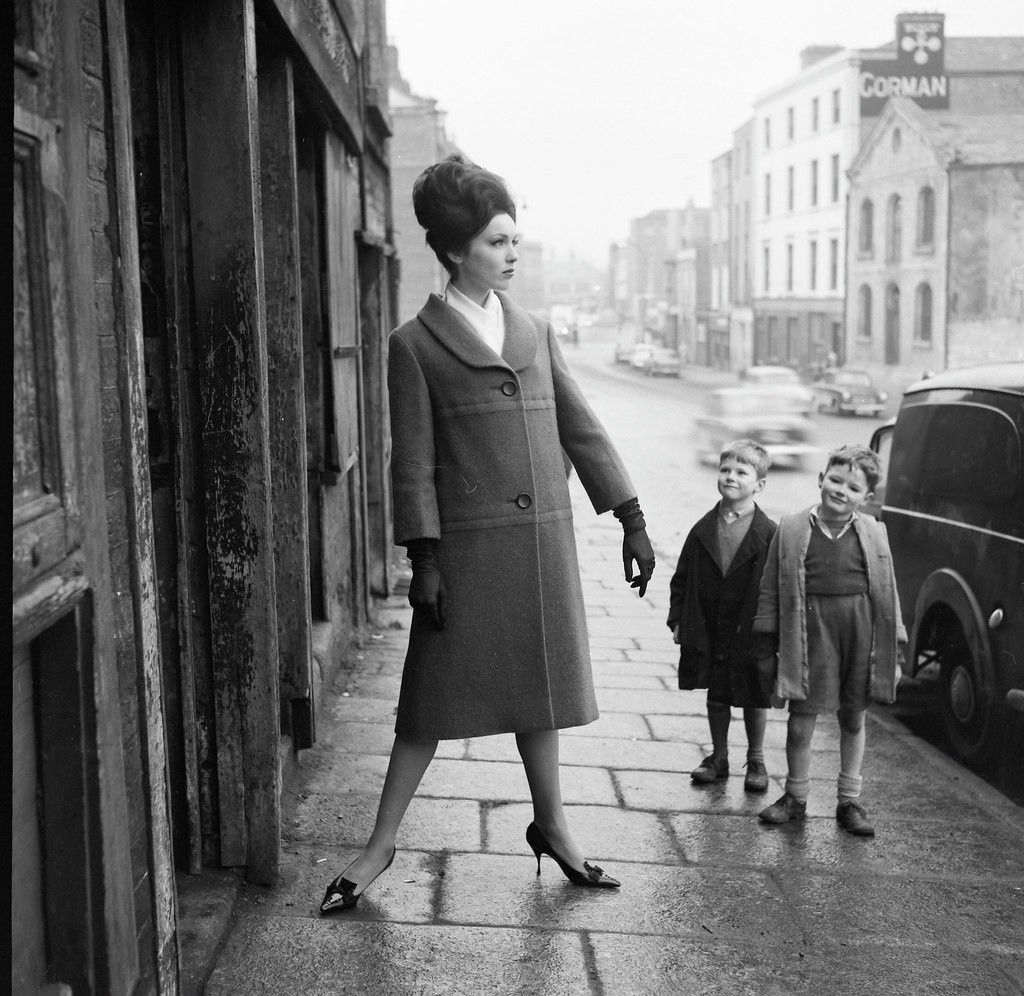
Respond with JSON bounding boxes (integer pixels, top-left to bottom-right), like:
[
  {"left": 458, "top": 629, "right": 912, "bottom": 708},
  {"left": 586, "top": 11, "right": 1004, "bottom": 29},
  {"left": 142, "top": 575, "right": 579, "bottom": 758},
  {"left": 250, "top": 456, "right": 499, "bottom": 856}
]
[
  {"left": 744, "top": 759, "right": 769, "bottom": 790},
  {"left": 690, "top": 754, "right": 729, "bottom": 783},
  {"left": 836, "top": 800, "right": 875, "bottom": 834},
  {"left": 757, "top": 793, "right": 807, "bottom": 824}
]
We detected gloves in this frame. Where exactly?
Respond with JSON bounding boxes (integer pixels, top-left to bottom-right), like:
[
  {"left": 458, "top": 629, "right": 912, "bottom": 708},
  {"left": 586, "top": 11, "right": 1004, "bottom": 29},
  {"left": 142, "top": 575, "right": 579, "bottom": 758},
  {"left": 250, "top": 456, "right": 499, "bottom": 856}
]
[
  {"left": 406, "top": 543, "right": 448, "bottom": 630},
  {"left": 616, "top": 504, "right": 656, "bottom": 598}
]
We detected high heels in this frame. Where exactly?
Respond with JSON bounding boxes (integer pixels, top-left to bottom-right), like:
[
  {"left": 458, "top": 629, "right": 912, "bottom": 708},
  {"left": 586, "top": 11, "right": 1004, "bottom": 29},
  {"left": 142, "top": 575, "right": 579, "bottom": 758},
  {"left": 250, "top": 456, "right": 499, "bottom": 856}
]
[
  {"left": 525, "top": 822, "right": 621, "bottom": 887},
  {"left": 320, "top": 844, "right": 396, "bottom": 914}
]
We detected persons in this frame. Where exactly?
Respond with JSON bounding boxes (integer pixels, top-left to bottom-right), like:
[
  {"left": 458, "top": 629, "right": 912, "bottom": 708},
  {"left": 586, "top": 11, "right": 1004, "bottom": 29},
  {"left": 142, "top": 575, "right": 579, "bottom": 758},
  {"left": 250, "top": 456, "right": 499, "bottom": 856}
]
[
  {"left": 666, "top": 439, "right": 785, "bottom": 791},
  {"left": 318, "top": 155, "right": 654, "bottom": 912},
  {"left": 754, "top": 447, "right": 906, "bottom": 836}
]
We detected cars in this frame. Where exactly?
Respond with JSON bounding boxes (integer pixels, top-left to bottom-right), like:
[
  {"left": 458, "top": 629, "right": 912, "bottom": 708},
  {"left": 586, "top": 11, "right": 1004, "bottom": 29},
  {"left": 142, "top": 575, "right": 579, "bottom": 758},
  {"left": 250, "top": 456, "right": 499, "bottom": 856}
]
[
  {"left": 550, "top": 310, "right": 820, "bottom": 473},
  {"left": 813, "top": 364, "right": 888, "bottom": 418},
  {"left": 865, "top": 361, "right": 1024, "bottom": 764}
]
